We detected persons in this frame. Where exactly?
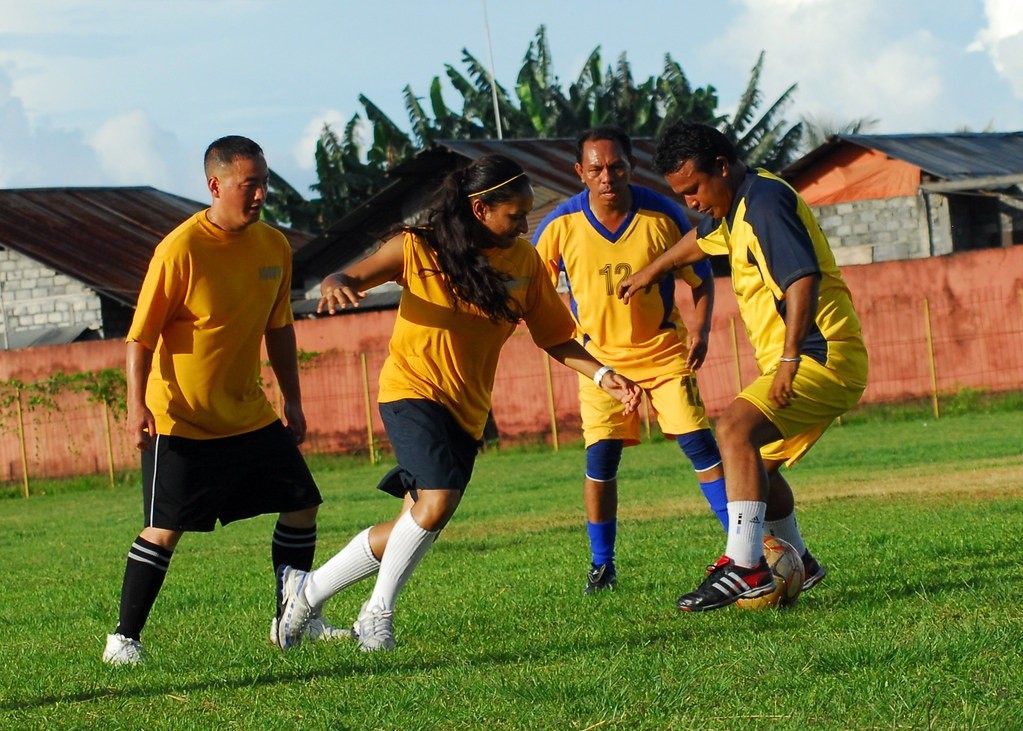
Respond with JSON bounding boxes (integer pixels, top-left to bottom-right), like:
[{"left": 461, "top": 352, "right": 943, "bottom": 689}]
[
  {"left": 100, "top": 135, "right": 322, "bottom": 668},
  {"left": 266, "top": 154, "right": 642, "bottom": 652},
  {"left": 615, "top": 120, "right": 870, "bottom": 613},
  {"left": 531, "top": 124, "right": 730, "bottom": 593}
]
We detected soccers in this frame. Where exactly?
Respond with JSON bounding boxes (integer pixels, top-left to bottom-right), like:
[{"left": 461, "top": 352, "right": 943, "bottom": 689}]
[{"left": 732, "top": 533, "right": 808, "bottom": 613}]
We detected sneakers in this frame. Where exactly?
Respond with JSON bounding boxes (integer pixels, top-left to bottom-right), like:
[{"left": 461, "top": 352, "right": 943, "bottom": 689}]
[
  {"left": 583, "top": 561, "right": 616, "bottom": 594},
  {"left": 801, "top": 547, "right": 825, "bottom": 591},
  {"left": 102, "top": 632, "right": 149, "bottom": 666},
  {"left": 352, "top": 597, "right": 396, "bottom": 654},
  {"left": 275, "top": 564, "right": 318, "bottom": 652},
  {"left": 270, "top": 617, "right": 352, "bottom": 645},
  {"left": 678, "top": 555, "right": 777, "bottom": 613}
]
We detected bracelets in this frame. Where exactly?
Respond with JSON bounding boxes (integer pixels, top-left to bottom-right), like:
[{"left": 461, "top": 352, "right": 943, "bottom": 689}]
[
  {"left": 593, "top": 365, "right": 614, "bottom": 388},
  {"left": 779, "top": 355, "right": 802, "bottom": 363}
]
